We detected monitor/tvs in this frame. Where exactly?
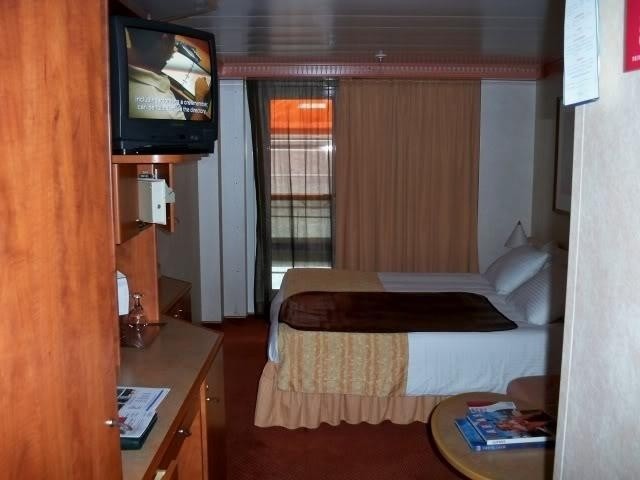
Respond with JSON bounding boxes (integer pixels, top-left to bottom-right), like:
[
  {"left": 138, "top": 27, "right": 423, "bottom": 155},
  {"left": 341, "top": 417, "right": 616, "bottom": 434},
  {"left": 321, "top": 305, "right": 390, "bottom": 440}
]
[{"left": 110, "top": 13, "right": 219, "bottom": 156}]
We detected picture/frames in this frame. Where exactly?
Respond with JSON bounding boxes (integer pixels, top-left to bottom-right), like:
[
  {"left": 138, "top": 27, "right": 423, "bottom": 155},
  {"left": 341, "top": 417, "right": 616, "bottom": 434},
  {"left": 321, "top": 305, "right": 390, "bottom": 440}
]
[{"left": 552, "top": 96, "right": 574, "bottom": 215}]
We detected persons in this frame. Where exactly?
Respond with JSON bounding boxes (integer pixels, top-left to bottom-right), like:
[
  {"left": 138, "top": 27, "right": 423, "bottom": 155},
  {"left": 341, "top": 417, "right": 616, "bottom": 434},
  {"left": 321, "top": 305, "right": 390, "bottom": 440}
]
[{"left": 125, "top": 26, "right": 212, "bottom": 121}]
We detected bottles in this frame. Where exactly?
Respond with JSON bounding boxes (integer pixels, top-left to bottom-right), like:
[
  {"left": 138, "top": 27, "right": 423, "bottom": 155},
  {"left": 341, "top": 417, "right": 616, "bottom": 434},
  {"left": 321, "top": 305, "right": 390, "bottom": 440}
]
[{"left": 128, "top": 293, "right": 149, "bottom": 351}]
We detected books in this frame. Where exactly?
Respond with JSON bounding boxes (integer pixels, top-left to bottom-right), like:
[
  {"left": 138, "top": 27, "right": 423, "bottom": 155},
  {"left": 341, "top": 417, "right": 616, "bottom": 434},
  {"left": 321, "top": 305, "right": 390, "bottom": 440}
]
[
  {"left": 160, "top": 51, "right": 212, "bottom": 100},
  {"left": 120, "top": 411, "right": 159, "bottom": 450},
  {"left": 453, "top": 398, "right": 556, "bottom": 452}
]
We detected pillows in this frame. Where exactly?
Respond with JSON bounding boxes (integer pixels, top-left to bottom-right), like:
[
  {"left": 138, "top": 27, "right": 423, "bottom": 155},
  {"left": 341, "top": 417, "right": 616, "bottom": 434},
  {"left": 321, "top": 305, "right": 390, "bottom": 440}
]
[
  {"left": 484, "top": 241, "right": 550, "bottom": 298},
  {"left": 507, "top": 264, "right": 567, "bottom": 324}
]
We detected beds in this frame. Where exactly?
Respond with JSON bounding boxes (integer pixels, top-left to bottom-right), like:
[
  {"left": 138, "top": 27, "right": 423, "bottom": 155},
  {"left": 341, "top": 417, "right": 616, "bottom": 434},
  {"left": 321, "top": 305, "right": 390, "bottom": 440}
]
[{"left": 254, "top": 244, "right": 567, "bottom": 430}]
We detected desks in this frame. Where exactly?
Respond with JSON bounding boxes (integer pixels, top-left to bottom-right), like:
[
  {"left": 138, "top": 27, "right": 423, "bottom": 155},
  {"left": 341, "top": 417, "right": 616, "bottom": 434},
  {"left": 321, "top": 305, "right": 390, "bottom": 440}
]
[{"left": 428, "top": 391, "right": 555, "bottom": 479}]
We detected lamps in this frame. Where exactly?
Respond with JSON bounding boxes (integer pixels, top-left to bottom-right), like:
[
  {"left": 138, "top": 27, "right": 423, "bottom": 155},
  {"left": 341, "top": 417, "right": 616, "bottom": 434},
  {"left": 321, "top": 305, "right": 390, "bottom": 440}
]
[{"left": 504, "top": 220, "right": 527, "bottom": 248}]
